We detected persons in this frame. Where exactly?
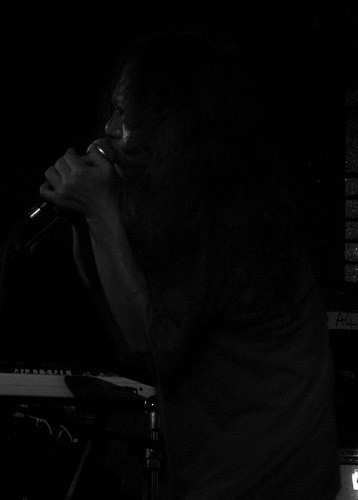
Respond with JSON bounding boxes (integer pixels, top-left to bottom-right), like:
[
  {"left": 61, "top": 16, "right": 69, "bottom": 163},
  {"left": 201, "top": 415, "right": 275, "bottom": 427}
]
[{"left": 39, "top": 26, "right": 343, "bottom": 499}]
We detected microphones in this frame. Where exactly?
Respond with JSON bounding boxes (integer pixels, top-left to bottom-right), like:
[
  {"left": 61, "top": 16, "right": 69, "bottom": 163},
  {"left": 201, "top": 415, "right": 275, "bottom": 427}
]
[{"left": 28, "top": 138, "right": 115, "bottom": 221}]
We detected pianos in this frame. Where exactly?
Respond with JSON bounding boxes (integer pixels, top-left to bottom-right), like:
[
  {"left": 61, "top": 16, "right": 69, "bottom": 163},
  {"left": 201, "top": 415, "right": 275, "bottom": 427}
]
[{"left": 0, "top": 366, "right": 157, "bottom": 408}]
